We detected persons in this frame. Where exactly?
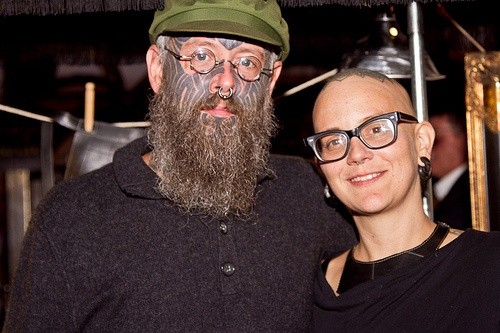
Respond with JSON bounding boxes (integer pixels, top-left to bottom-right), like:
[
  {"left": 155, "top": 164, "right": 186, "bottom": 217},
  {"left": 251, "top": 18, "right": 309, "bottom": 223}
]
[
  {"left": 302, "top": 67, "right": 500, "bottom": 333},
  {"left": 409, "top": 103, "right": 500, "bottom": 235},
  {"left": 2, "top": 1, "right": 361, "bottom": 333}
]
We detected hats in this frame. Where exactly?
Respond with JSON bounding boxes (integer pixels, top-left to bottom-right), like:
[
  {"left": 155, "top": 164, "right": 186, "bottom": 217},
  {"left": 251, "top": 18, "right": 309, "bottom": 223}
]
[{"left": 148, "top": 0, "right": 290, "bottom": 62}]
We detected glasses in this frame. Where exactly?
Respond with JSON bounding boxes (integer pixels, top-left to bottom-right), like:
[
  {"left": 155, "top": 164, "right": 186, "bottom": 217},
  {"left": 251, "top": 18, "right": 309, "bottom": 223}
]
[
  {"left": 302, "top": 111, "right": 419, "bottom": 163},
  {"left": 163, "top": 45, "right": 273, "bottom": 81}
]
